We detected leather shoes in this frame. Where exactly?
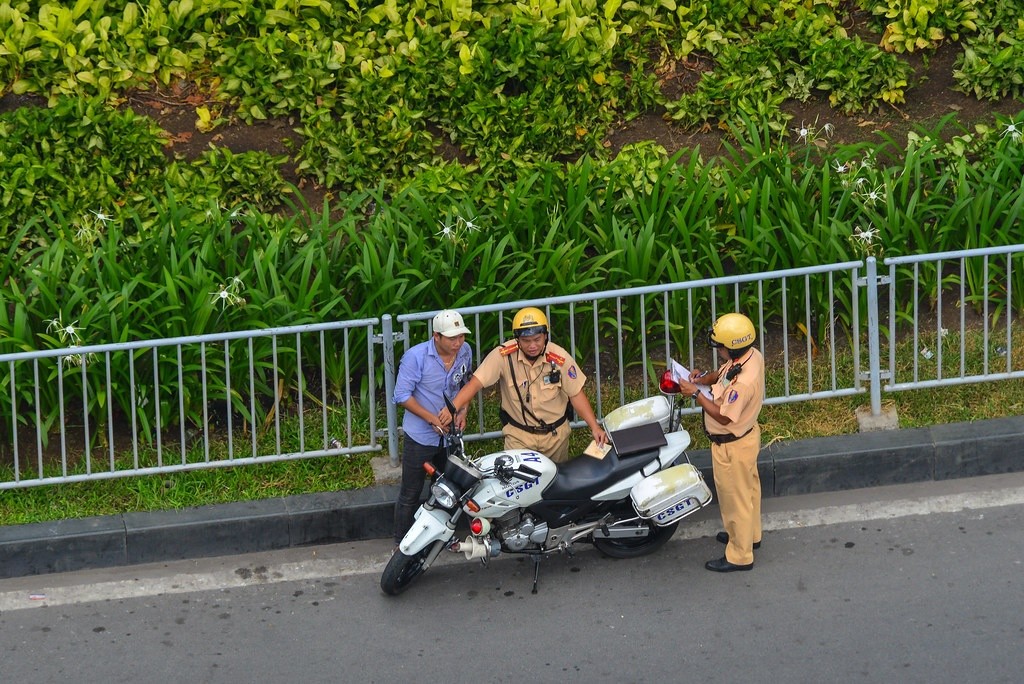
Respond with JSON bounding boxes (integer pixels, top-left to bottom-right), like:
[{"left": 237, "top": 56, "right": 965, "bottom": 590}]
[
  {"left": 705, "top": 555, "right": 753, "bottom": 573},
  {"left": 716, "top": 532, "right": 761, "bottom": 549}
]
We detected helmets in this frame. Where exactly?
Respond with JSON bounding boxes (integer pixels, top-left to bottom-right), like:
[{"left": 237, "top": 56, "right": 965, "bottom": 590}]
[
  {"left": 513, "top": 307, "right": 549, "bottom": 336},
  {"left": 706, "top": 313, "right": 756, "bottom": 350}
]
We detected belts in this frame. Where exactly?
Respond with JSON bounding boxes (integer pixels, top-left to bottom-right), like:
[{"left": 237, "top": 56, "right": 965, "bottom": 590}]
[
  {"left": 509, "top": 413, "right": 567, "bottom": 435},
  {"left": 702, "top": 407, "right": 753, "bottom": 447}
]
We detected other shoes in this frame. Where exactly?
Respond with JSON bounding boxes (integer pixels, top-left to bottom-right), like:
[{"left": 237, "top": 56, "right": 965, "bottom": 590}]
[{"left": 445, "top": 537, "right": 463, "bottom": 553}]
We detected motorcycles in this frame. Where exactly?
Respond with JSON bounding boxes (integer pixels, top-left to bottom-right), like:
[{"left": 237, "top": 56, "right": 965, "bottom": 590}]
[{"left": 380, "top": 369, "right": 714, "bottom": 596}]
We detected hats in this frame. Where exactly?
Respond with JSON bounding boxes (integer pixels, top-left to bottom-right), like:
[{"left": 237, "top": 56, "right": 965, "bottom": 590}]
[{"left": 433, "top": 309, "right": 472, "bottom": 338}]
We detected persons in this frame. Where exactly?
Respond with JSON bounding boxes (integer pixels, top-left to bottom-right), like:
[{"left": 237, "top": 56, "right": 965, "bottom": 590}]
[
  {"left": 679, "top": 312, "right": 765, "bottom": 572},
  {"left": 391, "top": 309, "right": 472, "bottom": 556},
  {"left": 438, "top": 308, "right": 609, "bottom": 464}
]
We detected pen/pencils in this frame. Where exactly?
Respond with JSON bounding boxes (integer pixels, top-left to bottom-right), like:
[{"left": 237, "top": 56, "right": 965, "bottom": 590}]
[{"left": 691, "top": 370, "right": 708, "bottom": 382}]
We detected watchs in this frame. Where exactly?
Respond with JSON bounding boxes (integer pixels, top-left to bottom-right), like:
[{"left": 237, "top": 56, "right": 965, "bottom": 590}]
[{"left": 691, "top": 389, "right": 701, "bottom": 400}]
